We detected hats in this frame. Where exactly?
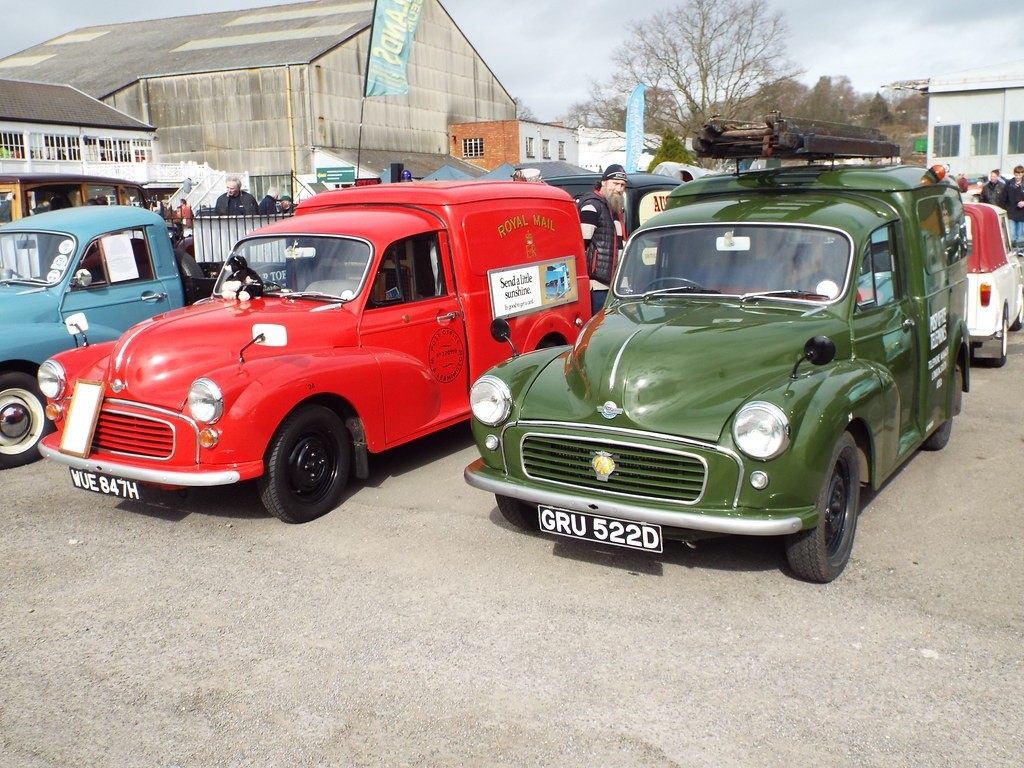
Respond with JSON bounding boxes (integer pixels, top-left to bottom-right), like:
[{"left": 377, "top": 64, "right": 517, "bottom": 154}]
[
  {"left": 275, "top": 196, "right": 291, "bottom": 202},
  {"left": 600, "top": 164, "right": 627, "bottom": 184}
]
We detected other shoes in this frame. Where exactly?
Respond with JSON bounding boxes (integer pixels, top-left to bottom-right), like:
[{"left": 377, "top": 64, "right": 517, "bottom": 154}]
[{"left": 1017, "top": 249, "right": 1023, "bottom": 257}]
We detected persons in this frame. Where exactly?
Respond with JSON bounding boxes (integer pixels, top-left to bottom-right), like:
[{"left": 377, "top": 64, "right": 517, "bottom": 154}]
[
  {"left": 37, "top": 191, "right": 109, "bottom": 215},
  {"left": 279, "top": 196, "right": 299, "bottom": 213},
  {"left": 143, "top": 195, "right": 209, "bottom": 240},
  {"left": 576, "top": 164, "right": 627, "bottom": 317},
  {"left": 257, "top": 189, "right": 280, "bottom": 214},
  {"left": 1001, "top": 165, "right": 1024, "bottom": 256},
  {"left": 184, "top": 177, "right": 199, "bottom": 193},
  {"left": 216, "top": 176, "right": 259, "bottom": 216},
  {"left": 980, "top": 170, "right": 1005, "bottom": 207}
]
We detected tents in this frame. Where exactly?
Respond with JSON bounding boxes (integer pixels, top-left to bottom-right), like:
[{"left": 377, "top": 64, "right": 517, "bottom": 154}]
[{"left": 375, "top": 162, "right": 523, "bottom": 185}]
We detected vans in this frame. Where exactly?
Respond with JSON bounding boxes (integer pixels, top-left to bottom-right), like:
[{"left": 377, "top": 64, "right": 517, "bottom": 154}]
[{"left": 0, "top": 172, "right": 150, "bottom": 229}]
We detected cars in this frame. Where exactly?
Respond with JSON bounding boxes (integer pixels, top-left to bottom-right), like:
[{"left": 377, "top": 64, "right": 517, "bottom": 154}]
[
  {"left": 963, "top": 202, "right": 1024, "bottom": 369},
  {"left": 0, "top": 205, "right": 203, "bottom": 470}
]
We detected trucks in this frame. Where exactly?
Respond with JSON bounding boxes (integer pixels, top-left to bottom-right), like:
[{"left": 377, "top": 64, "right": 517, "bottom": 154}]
[
  {"left": 38, "top": 179, "right": 592, "bottom": 525},
  {"left": 462, "top": 164, "right": 971, "bottom": 583}
]
